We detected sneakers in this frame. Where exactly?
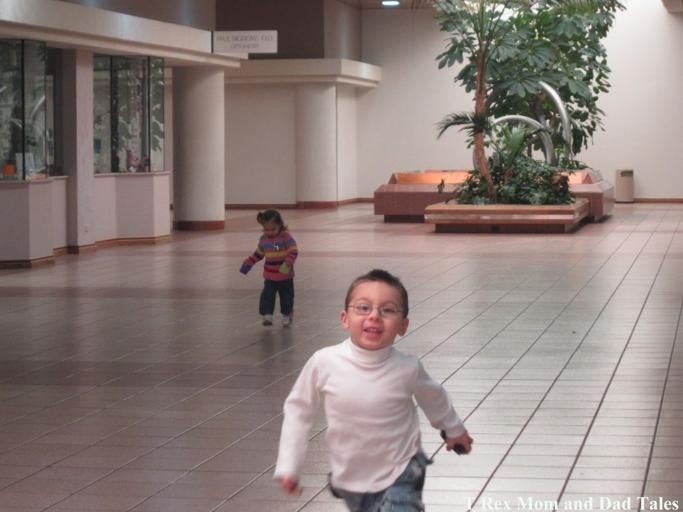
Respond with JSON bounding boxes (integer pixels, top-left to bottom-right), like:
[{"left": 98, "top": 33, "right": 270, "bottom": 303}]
[{"left": 263, "top": 310, "right": 293, "bottom": 327}]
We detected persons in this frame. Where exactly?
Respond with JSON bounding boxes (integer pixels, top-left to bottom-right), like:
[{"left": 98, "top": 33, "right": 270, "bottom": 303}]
[
  {"left": 271, "top": 269, "right": 473, "bottom": 512},
  {"left": 240, "top": 209, "right": 298, "bottom": 328}
]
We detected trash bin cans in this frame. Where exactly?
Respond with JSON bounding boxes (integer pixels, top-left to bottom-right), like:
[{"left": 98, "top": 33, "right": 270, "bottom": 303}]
[{"left": 614, "top": 169, "right": 634, "bottom": 203}]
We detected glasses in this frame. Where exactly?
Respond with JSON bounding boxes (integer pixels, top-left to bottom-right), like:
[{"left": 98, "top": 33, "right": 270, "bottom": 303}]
[{"left": 345, "top": 302, "right": 403, "bottom": 316}]
